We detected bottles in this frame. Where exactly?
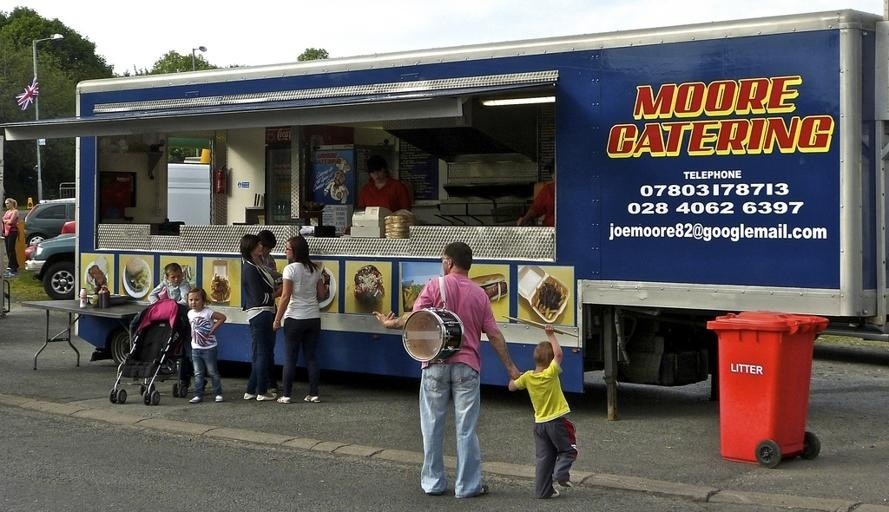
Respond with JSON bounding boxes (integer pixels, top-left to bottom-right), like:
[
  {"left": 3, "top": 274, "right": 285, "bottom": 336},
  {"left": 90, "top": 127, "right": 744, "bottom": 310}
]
[
  {"left": 79, "top": 286, "right": 111, "bottom": 310},
  {"left": 271, "top": 159, "right": 292, "bottom": 223}
]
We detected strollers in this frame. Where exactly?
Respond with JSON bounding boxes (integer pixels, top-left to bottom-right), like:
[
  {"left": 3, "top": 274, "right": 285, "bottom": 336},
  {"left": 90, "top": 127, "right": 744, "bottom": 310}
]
[{"left": 107, "top": 298, "right": 192, "bottom": 407}]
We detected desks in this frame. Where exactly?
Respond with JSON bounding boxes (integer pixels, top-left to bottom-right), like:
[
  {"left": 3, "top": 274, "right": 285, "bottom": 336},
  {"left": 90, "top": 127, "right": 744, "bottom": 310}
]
[{"left": 22, "top": 300, "right": 148, "bottom": 385}]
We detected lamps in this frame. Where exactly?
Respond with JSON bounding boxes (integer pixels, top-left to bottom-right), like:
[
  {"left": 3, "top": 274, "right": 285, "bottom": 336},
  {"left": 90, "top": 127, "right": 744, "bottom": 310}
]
[{"left": 479, "top": 95, "right": 556, "bottom": 107}]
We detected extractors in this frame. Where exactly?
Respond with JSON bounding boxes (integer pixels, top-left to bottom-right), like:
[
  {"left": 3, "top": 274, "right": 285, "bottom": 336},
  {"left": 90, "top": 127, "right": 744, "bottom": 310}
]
[{"left": 382, "top": 95, "right": 539, "bottom": 161}]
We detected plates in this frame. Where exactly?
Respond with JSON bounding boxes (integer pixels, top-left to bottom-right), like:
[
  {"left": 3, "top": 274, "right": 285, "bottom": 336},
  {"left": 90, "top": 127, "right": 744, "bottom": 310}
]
[
  {"left": 318, "top": 266, "right": 336, "bottom": 310},
  {"left": 123, "top": 260, "right": 151, "bottom": 299},
  {"left": 210, "top": 275, "right": 232, "bottom": 303},
  {"left": 85, "top": 261, "right": 110, "bottom": 288}
]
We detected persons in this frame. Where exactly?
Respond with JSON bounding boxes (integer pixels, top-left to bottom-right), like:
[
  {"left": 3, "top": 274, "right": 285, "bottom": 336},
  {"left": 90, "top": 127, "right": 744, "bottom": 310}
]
[
  {"left": 354, "top": 154, "right": 410, "bottom": 217},
  {"left": 149, "top": 230, "right": 327, "bottom": 404},
  {"left": 370, "top": 239, "right": 523, "bottom": 499},
  {"left": 507, "top": 324, "right": 581, "bottom": 500},
  {"left": 0, "top": 197, "right": 19, "bottom": 278},
  {"left": 515, "top": 155, "right": 555, "bottom": 226}
]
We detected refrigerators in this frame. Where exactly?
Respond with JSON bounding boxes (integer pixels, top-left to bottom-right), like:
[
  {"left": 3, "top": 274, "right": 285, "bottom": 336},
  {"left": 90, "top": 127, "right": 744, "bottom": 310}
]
[
  {"left": 307, "top": 143, "right": 389, "bottom": 237},
  {"left": 264, "top": 145, "right": 291, "bottom": 226}
]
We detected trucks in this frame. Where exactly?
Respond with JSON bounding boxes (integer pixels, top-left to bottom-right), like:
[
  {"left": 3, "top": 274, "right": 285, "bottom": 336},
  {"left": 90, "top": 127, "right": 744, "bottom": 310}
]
[{"left": 3, "top": 5, "right": 889, "bottom": 382}]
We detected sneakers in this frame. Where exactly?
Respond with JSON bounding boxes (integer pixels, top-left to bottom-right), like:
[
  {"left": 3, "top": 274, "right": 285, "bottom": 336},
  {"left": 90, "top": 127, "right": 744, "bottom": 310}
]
[
  {"left": 551, "top": 489, "right": 561, "bottom": 497},
  {"left": 304, "top": 395, "right": 321, "bottom": 403},
  {"left": 257, "top": 393, "right": 277, "bottom": 401},
  {"left": 482, "top": 484, "right": 490, "bottom": 494},
  {"left": 189, "top": 397, "right": 201, "bottom": 403},
  {"left": 215, "top": 395, "right": 224, "bottom": 403},
  {"left": 244, "top": 392, "right": 257, "bottom": 400},
  {"left": 277, "top": 396, "right": 291, "bottom": 404},
  {"left": 559, "top": 481, "right": 573, "bottom": 488}
]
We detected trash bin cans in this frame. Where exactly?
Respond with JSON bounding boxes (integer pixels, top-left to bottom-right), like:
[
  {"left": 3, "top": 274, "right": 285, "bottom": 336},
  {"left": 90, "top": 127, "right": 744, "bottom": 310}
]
[{"left": 706, "top": 311, "right": 828, "bottom": 468}]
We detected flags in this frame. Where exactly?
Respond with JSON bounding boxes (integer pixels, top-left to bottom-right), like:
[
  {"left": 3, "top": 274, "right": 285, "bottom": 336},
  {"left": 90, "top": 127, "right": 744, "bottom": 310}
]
[{"left": 14, "top": 74, "right": 42, "bottom": 113}]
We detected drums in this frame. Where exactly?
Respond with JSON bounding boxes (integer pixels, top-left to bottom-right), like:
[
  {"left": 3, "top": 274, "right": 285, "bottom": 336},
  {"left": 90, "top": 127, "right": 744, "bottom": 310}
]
[{"left": 404, "top": 308, "right": 463, "bottom": 361}]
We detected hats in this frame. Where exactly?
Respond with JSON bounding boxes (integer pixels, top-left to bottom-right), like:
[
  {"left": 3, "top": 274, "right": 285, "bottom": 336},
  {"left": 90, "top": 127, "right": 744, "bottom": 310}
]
[
  {"left": 259, "top": 230, "right": 277, "bottom": 248},
  {"left": 367, "top": 156, "right": 387, "bottom": 172}
]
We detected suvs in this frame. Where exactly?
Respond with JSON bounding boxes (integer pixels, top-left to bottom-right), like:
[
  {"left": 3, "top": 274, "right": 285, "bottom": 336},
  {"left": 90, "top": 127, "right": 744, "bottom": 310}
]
[{"left": 25, "top": 198, "right": 76, "bottom": 245}]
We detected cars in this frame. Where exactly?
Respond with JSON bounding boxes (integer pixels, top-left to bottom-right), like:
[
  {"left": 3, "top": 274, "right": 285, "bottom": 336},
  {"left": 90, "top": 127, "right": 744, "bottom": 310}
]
[{"left": 24, "top": 218, "right": 76, "bottom": 300}]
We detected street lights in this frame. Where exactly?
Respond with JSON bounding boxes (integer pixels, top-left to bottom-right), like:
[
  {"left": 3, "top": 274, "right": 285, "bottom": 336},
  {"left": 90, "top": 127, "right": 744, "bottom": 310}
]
[
  {"left": 27, "top": 30, "right": 66, "bottom": 199},
  {"left": 192, "top": 46, "right": 207, "bottom": 71}
]
